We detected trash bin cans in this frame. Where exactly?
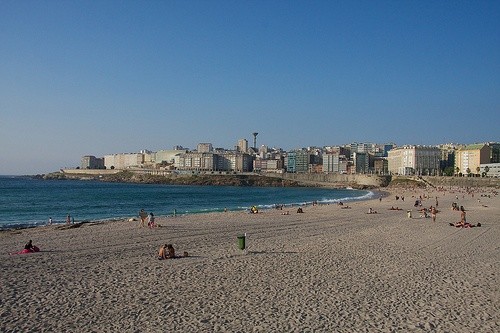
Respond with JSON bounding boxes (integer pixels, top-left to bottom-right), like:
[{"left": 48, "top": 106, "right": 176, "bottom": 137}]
[{"left": 237, "top": 236, "right": 246, "bottom": 250}]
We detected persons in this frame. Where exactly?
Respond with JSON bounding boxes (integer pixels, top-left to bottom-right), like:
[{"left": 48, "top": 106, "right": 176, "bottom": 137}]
[
  {"left": 49, "top": 217, "right": 52, "bottom": 224},
  {"left": 379, "top": 194, "right": 382, "bottom": 202},
  {"left": 451, "top": 202, "right": 460, "bottom": 211},
  {"left": 422, "top": 205, "right": 437, "bottom": 222},
  {"left": 251, "top": 205, "right": 257, "bottom": 214},
  {"left": 24, "top": 240, "right": 34, "bottom": 253},
  {"left": 297, "top": 208, "right": 303, "bottom": 213},
  {"left": 368, "top": 207, "right": 372, "bottom": 214},
  {"left": 395, "top": 195, "right": 404, "bottom": 200},
  {"left": 461, "top": 207, "right": 466, "bottom": 228},
  {"left": 419, "top": 196, "right": 423, "bottom": 207},
  {"left": 158, "top": 244, "right": 176, "bottom": 260},
  {"left": 435, "top": 196, "right": 439, "bottom": 205},
  {"left": 72, "top": 216, "right": 75, "bottom": 224},
  {"left": 275, "top": 202, "right": 287, "bottom": 210},
  {"left": 438, "top": 186, "right": 499, "bottom": 199},
  {"left": 138, "top": 208, "right": 154, "bottom": 228}
]
